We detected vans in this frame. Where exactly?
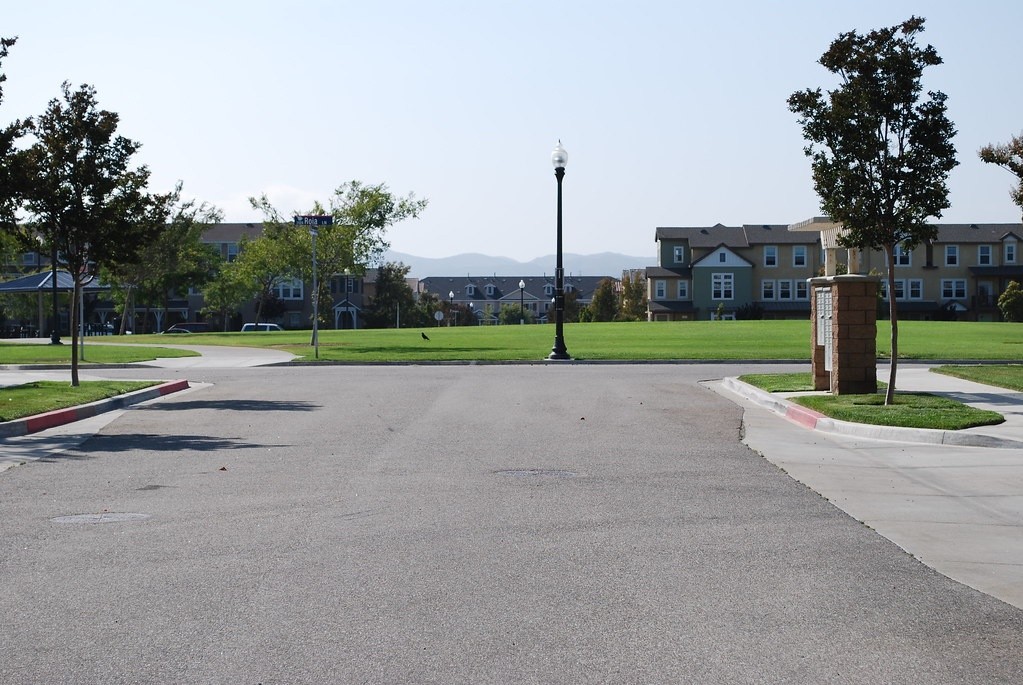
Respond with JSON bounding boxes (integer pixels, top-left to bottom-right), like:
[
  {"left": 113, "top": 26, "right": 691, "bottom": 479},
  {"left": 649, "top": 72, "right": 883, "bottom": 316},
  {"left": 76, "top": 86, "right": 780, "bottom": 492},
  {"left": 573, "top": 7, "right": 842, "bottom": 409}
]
[{"left": 240, "top": 322, "right": 283, "bottom": 332}]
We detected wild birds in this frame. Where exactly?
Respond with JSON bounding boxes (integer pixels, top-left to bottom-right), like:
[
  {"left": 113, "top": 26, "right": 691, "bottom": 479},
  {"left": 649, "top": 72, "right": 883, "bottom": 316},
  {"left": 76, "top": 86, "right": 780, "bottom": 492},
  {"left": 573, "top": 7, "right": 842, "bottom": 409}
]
[{"left": 421, "top": 332, "right": 430, "bottom": 340}]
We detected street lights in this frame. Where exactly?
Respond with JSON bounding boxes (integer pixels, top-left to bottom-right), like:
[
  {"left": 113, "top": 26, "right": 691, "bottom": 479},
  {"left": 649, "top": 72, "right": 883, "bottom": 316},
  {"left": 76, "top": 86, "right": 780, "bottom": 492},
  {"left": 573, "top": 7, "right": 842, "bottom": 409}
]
[
  {"left": 546, "top": 138, "right": 568, "bottom": 359},
  {"left": 35, "top": 235, "right": 43, "bottom": 337},
  {"left": 449, "top": 291, "right": 455, "bottom": 326},
  {"left": 519, "top": 279, "right": 525, "bottom": 324},
  {"left": 344, "top": 267, "right": 350, "bottom": 329},
  {"left": 470, "top": 302, "right": 474, "bottom": 326}
]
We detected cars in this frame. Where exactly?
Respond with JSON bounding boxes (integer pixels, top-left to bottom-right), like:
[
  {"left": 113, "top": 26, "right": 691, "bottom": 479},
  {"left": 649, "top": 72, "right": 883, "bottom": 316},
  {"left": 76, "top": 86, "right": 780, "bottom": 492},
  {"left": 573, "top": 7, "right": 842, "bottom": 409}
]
[{"left": 160, "top": 328, "right": 191, "bottom": 334}]
